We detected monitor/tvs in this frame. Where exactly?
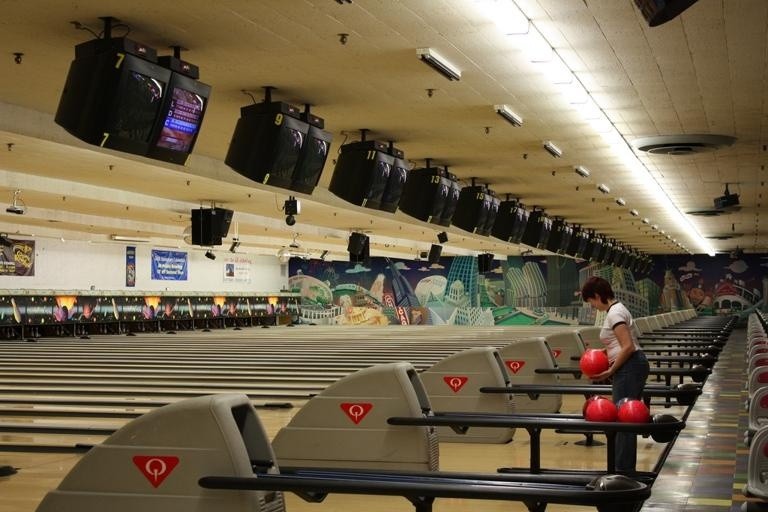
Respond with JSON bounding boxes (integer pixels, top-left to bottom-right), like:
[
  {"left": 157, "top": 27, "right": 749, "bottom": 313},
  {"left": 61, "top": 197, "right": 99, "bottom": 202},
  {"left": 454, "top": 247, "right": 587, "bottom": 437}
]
[
  {"left": 224, "top": 113, "right": 333, "bottom": 195},
  {"left": 327, "top": 150, "right": 652, "bottom": 275},
  {"left": 53, "top": 53, "right": 211, "bottom": 166}
]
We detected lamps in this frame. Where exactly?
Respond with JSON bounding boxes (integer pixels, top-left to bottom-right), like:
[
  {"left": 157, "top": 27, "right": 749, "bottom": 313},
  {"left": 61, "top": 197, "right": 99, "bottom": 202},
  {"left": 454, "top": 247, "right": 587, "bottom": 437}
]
[{"left": 411, "top": 46, "right": 611, "bottom": 195}]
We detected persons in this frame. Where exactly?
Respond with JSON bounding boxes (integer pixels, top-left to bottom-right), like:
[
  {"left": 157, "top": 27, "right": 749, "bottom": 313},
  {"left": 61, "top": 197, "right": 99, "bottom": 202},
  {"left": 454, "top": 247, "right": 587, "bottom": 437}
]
[{"left": 581, "top": 276, "right": 650, "bottom": 470}]
